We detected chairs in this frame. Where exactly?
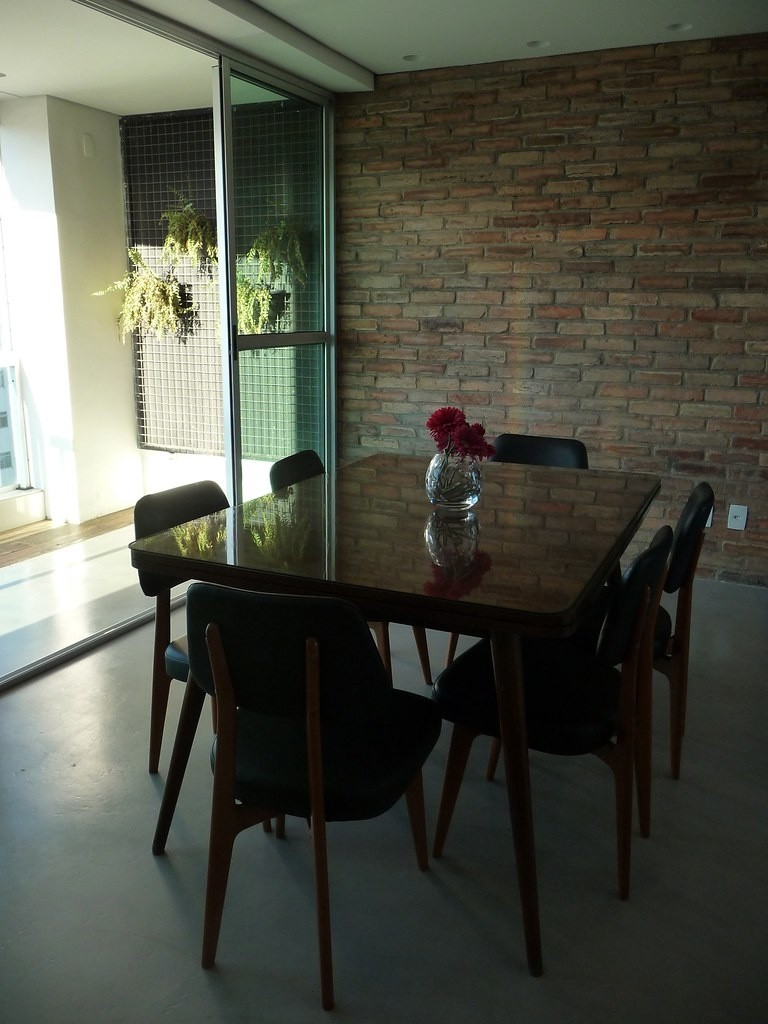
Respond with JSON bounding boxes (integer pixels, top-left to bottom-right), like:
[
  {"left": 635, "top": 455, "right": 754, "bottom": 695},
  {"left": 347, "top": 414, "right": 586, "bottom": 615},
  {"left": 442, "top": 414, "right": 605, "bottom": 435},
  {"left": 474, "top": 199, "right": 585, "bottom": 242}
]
[
  {"left": 269, "top": 449, "right": 326, "bottom": 492},
  {"left": 429, "top": 523, "right": 673, "bottom": 904},
  {"left": 487, "top": 434, "right": 589, "bottom": 470},
  {"left": 133, "top": 480, "right": 218, "bottom": 772},
  {"left": 184, "top": 581, "right": 443, "bottom": 1012},
  {"left": 566, "top": 480, "right": 715, "bottom": 782}
]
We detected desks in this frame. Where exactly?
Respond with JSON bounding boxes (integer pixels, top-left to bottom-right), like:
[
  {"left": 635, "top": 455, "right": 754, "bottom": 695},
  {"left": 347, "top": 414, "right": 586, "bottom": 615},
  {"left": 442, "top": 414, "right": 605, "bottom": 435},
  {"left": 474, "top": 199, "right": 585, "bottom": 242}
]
[{"left": 126, "top": 448, "right": 664, "bottom": 976}]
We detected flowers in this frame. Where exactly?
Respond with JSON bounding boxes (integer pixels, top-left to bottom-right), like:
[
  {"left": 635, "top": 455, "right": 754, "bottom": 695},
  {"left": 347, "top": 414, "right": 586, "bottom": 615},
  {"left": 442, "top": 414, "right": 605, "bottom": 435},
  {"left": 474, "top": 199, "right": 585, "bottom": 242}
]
[
  {"left": 425, "top": 405, "right": 496, "bottom": 464},
  {"left": 420, "top": 548, "right": 495, "bottom": 601}
]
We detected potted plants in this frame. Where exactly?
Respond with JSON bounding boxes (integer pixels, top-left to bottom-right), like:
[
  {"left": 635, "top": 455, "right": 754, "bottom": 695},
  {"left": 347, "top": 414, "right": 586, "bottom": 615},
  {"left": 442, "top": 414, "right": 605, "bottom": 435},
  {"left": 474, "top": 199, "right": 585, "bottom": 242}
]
[{"left": 229, "top": 271, "right": 281, "bottom": 337}]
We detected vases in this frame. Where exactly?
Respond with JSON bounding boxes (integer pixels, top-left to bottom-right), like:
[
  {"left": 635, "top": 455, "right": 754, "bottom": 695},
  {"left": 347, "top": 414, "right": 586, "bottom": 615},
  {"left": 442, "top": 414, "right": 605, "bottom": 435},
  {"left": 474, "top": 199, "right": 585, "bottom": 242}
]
[
  {"left": 421, "top": 507, "right": 480, "bottom": 565},
  {"left": 425, "top": 451, "right": 488, "bottom": 512}
]
